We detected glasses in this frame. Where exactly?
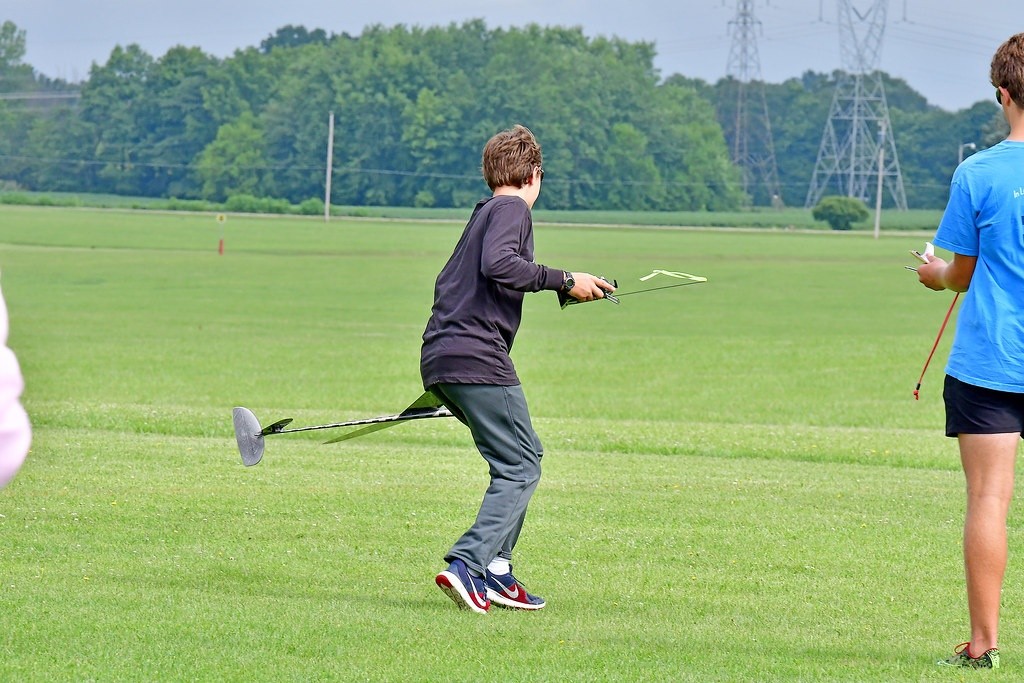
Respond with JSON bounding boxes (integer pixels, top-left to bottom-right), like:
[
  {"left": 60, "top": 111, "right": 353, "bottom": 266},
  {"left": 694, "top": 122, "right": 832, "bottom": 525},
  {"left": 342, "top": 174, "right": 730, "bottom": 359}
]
[
  {"left": 539, "top": 167, "right": 544, "bottom": 181},
  {"left": 996, "top": 88, "right": 1002, "bottom": 104}
]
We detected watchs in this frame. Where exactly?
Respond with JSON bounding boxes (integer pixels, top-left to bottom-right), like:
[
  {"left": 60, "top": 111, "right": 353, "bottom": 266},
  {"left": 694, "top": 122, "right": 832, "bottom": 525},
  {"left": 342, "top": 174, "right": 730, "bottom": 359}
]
[{"left": 561, "top": 272, "right": 575, "bottom": 293}]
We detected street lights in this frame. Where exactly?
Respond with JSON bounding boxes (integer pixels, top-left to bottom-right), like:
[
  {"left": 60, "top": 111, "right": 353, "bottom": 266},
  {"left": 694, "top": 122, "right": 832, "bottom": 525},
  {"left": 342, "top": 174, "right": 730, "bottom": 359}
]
[{"left": 958, "top": 142, "right": 976, "bottom": 164}]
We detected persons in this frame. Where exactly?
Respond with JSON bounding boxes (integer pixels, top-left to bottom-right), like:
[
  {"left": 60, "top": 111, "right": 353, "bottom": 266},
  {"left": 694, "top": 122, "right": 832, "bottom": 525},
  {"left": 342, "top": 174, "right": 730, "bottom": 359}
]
[
  {"left": 420, "top": 124, "right": 615, "bottom": 613},
  {"left": 918, "top": 33, "right": 1024, "bottom": 670}
]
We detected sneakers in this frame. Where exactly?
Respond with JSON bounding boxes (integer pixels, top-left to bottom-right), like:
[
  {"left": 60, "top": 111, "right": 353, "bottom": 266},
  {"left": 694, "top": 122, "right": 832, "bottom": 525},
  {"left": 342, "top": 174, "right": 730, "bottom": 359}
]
[
  {"left": 435, "top": 559, "right": 491, "bottom": 614},
  {"left": 936, "top": 642, "right": 1000, "bottom": 671},
  {"left": 485, "top": 564, "right": 546, "bottom": 609}
]
